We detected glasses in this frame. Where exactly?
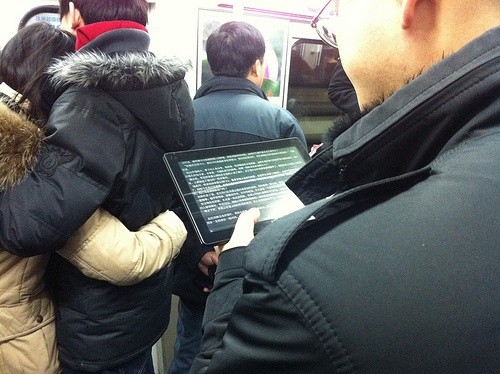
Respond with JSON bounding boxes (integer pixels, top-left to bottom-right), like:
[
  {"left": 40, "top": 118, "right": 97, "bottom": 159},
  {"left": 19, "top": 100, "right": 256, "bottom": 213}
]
[{"left": 310, "top": 0, "right": 338, "bottom": 49}]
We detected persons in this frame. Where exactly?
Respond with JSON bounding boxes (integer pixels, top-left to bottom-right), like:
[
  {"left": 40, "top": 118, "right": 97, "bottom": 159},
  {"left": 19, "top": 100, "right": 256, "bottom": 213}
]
[
  {"left": 1, "top": 0, "right": 209, "bottom": 374},
  {"left": 0, "top": 23, "right": 191, "bottom": 374},
  {"left": 192, "top": 0, "right": 500, "bottom": 374},
  {"left": 328, "top": 55, "right": 364, "bottom": 120},
  {"left": 165, "top": 20, "right": 317, "bottom": 374}
]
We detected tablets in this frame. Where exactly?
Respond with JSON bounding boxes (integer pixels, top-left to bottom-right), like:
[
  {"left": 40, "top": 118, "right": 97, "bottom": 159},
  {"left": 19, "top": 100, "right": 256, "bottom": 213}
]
[{"left": 162, "top": 137, "right": 318, "bottom": 244}]
